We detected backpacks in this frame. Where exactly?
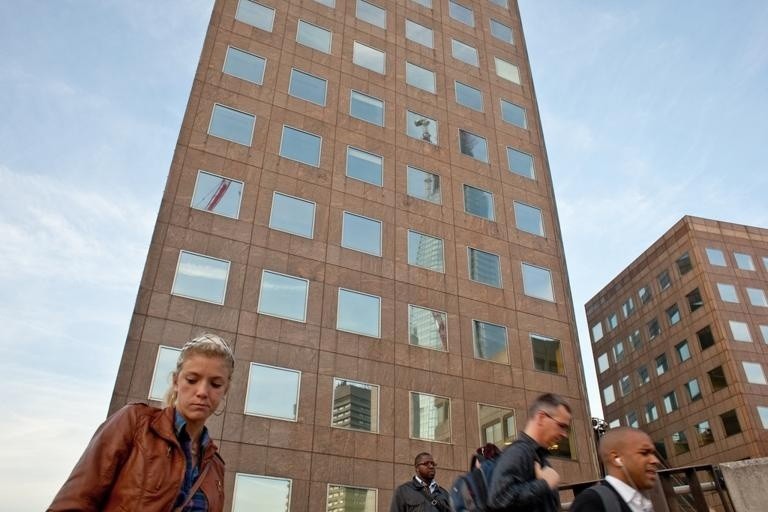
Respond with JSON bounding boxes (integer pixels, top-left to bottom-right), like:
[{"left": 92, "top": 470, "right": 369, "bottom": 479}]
[{"left": 448, "top": 440, "right": 541, "bottom": 512}]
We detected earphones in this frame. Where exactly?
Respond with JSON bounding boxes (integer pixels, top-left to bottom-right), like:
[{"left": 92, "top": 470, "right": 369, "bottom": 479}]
[{"left": 615, "top": 458, "right": 621, "bottom": 464}]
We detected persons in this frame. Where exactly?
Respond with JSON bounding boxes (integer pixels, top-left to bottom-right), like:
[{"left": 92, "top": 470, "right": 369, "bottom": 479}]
[
  {"left": 386, "top": 451, "right": 452, "bottom": 512},
  {"left": 480, "top": 390, "right": 574, "bottom": 512},
  {"left": 564, "top": 425, "right": 663, "bottom": 512},
  {"left": 45, "top": 331, "right": 237, "bottom": 512},
  {"left": 446, "top": 441, "right": 501, "bottom": 512}
]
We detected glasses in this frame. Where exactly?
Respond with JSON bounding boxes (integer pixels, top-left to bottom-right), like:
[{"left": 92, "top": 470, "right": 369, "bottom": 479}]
[
  {"left": 543, "top": 412, "right": 570, "bottom": 434},
  {"left": 416, "top": 461, "right": 437, "bottom": 467}
]
